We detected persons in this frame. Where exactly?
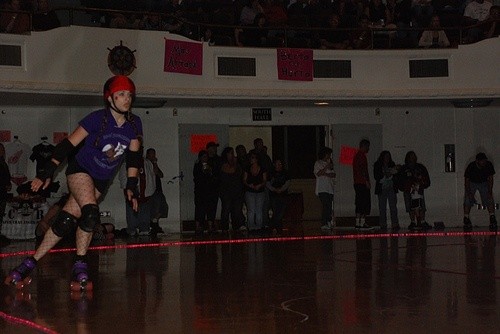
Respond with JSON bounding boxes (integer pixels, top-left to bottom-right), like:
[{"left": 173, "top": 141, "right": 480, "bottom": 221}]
[
  {"left": 193, "top": 138, "right": 292, "bottom": 237},
  {"left": 354, "top": 138, "right": 434, "bottom": 230},
  {"left": 463, "top": 152, "right": 498, "bottom": 230},
  {"left": 3, "top": 74, "right": 145, "bottom": 291},
  {"left": 32, "top": 147, "right": 165, "bottom": 251},
  {"left": 313, "top": 147, "right": 343, "bottom": 230}
]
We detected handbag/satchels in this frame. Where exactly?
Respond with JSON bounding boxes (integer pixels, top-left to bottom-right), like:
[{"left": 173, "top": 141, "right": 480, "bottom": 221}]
[
  {"left": 398, "top": 176, "right": 412, "bottom": 192},
  {"left": 375, "top": 181, "right": 382, "bottom": 195}
]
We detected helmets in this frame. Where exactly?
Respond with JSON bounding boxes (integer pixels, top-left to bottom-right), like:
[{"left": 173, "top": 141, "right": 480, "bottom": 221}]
[{"left": 104, "top": 75, "right": 136, "bottom": 99}]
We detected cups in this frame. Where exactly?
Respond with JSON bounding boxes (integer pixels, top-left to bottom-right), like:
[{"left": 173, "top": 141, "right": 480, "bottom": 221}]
[{"left": 202, "top": 162, "right": 207, "bottom": 169}]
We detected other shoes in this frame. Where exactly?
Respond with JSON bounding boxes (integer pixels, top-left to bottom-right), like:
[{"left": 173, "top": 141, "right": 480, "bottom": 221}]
[
  {"left": 355, "top": 222, "right": 372, "bottom": 228},
  {"left": 489, "top": 215, "right": 497, "bottom": 225},
  {"left": 392, "top": 225, "right": 400, "bottom": 229},
  {"left": 321, "top": 226, "right": 334, "bottom": 230},
  {"left": 381, "top": 224, "right": 387, "bottom": 229},
  {"left": 408, "top": 222, "right": 424, "bottom": 230},
  {"left": 151, "top": 223, "right": 165, "bottom": 233},
  {"left": 422, "top": 222, "right": 432, "bottom": 229},
  {"left": 463, "top": 216, "right": 472, "bottom": 225}
]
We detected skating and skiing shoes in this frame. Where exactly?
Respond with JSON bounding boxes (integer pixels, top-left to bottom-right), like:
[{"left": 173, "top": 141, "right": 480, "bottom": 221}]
[
  {"left": 70, "top": 260, "right": 94, "bottom": 291},
  {"left": 5, "top": 256, "right": 36, "bottom": 289}
]
